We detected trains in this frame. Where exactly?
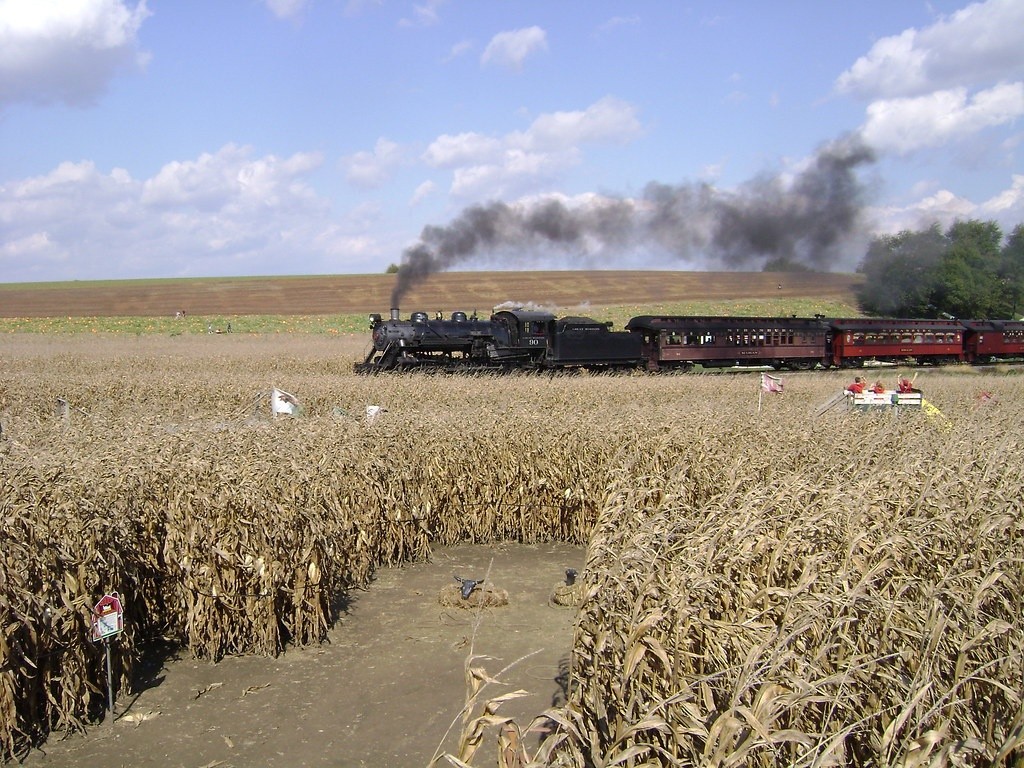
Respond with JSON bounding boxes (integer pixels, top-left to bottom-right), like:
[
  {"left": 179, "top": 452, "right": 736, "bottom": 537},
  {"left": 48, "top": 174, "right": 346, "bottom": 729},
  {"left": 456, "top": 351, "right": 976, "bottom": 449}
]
[{"left": 351, "top": 306, "right": 1024, "bottom": 378}]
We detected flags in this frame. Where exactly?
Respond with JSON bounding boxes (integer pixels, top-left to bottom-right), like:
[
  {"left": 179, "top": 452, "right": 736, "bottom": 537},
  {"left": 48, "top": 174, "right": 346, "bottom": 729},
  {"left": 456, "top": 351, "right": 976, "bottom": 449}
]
[
  {"left": 271, "top": 387, "right": 298, "bottom": 415},
  {"left": 760, "top": 372, "right": 786, "bottom": 394}
]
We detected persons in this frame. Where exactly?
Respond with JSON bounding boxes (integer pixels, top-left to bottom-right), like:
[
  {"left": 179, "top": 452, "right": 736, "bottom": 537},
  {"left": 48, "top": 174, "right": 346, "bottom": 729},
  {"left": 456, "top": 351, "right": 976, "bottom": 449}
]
[
  {"left": 898, "top": 372, "right": 919, "bottom": 393},
  {"left": 208, "top": 324, "right": 213, "bottom": 335},
  {"left": 175, "top": 312, "right": 181, "bottom": 320},
  {"left": 870, "top": 381, "right": 885, "bottom": 393},
  {"left": 227, "top": 323, "right": 232, "bottom": 333},
  {"left": 182, "top": 311, "right": 187, "bottom": 318},
  {"left": 848, "top": 377, "right": 867, "bottom": 395}
]
